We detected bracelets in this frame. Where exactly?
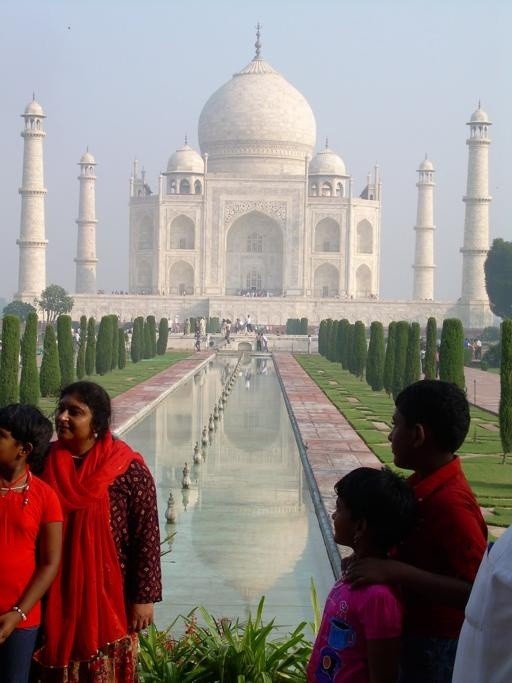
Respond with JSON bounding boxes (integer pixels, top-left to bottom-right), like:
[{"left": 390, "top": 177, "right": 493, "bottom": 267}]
[{"left": 10, "top": 605, "right": 30, "bottom": 620}]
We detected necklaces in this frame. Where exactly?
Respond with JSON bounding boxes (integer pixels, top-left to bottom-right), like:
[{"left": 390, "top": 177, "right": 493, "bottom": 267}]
[
  {"left": 68, "top": 452, "right": 89, "bottom": 462},
  {"left": 0, "top": 471, "right": 34, "bottom": 506}
]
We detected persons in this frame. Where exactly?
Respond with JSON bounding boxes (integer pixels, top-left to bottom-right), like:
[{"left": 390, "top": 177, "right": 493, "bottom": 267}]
[
  {"left": 1, "top": 401, "right": 66, "bottom": 683},
  {"left": 332, "top": 291, "right": 379, "bottom": 301},
  {"left": 96, "top": 286, "right": 166, "bottom": 296},
  {"left": 172, "top": 314, "right": 270, "bottom": 353},
  {"left": 55, "top": 327, "right": 97, "bottom": 346},
  {"left": 239, "top": 287, "right": 272, "bottom": 297},
  {"left": 419, "top": 336, "right": 482, "bottom": 375},
  {"left": 34, "top": 380, "right": 164, "bottom": 683},
  {"left": 337, "top": 374, "right": 491, "bottom": 682},
  {"left": 244, "top": 358, "right": 268, "bottom": 391},
  {"left": 305, "top": 465, "right": 424, "bottom": 682}
]
